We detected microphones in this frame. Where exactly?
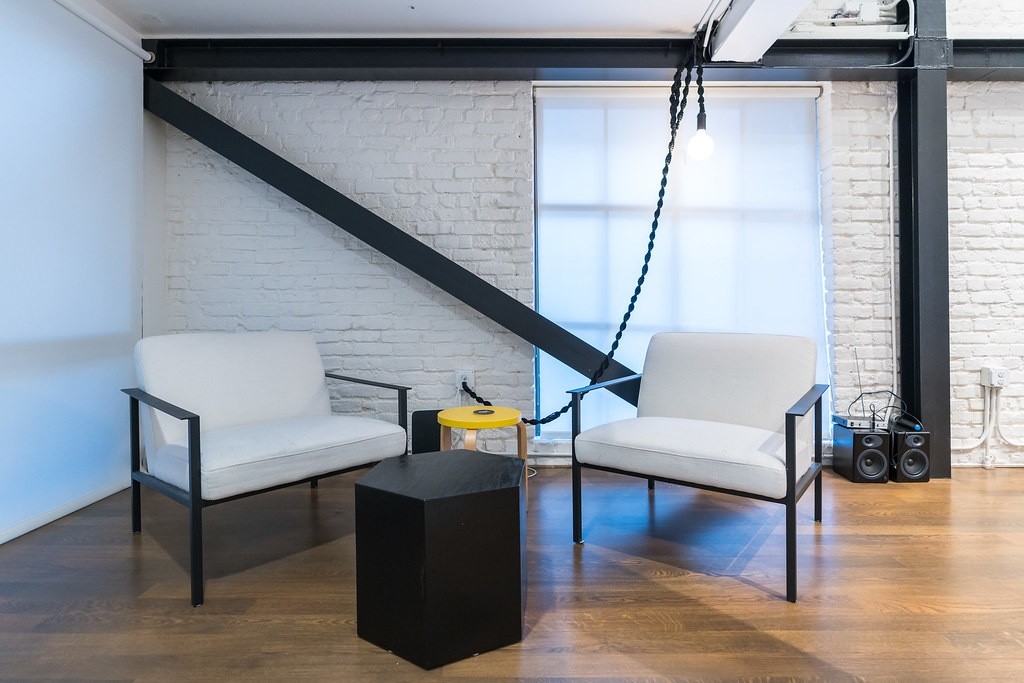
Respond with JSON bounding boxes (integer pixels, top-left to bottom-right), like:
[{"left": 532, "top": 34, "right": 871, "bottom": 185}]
[{"left": 890, "top": 414, "right": 921, "bottom": 431}]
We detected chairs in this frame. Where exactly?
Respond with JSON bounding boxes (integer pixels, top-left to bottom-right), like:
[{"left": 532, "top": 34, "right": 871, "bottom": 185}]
[
  {"left": 121, "top": 328, "right": 412, "bottom": 610},
  {"left": 565, "top": 333, "right": 827, "bottom": 604}
]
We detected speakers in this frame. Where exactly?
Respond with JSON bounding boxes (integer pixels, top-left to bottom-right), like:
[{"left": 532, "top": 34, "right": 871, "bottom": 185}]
[
  {"left": 887, "top": 426, "right": 931, "bottom": 482},
  {"left": 832, "top": 424, "right": 889, "bottom": 483}
]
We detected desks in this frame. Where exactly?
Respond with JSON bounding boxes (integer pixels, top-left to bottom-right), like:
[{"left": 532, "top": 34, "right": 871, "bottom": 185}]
[{"left": 355, "top": 449, "right": 523, "bottom": 669}]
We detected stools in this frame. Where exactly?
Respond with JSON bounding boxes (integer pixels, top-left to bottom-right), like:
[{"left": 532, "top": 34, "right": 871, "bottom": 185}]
[{"left": 436, "top": 405, "right": 528, "bottom": 514}]
